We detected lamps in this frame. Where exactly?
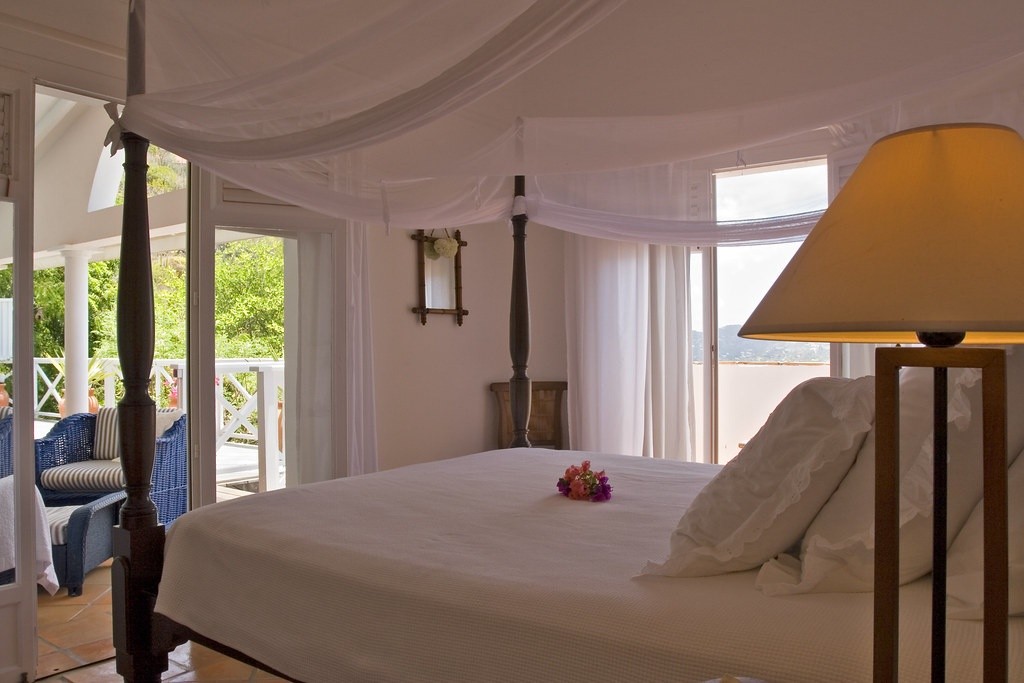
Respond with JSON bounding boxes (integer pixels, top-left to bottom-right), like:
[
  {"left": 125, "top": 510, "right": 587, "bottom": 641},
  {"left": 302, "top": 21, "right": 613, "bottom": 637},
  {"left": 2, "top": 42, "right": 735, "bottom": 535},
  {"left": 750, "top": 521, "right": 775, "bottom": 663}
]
[{"left": 735, "top": 123, "right": 1024, "bottom": 683}]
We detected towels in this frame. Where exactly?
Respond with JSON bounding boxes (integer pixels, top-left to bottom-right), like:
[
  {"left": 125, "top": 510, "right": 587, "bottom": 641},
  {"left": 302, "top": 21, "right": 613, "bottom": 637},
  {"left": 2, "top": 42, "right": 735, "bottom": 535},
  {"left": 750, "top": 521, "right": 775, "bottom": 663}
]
[{"left": 0, "top": 473, "right": 60, "bottom": 597}]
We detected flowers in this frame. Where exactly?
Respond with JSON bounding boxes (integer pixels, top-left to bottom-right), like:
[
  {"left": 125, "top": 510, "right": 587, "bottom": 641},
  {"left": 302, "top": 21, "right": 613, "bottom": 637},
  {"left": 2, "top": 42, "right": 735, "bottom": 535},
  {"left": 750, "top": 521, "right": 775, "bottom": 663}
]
[
  {"left": 165, "top": 375, "right": 221, "bottom": 398},
  {"left": 556, "top": 460, "right": 614, "bottom": 504}
]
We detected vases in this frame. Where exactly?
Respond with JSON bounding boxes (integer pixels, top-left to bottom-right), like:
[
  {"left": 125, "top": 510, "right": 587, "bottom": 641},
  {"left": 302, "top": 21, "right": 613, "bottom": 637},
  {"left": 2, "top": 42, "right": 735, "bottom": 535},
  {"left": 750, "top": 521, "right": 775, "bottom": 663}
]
[{"left": 169, "top": 395, "right": 179, "bottom": 409}]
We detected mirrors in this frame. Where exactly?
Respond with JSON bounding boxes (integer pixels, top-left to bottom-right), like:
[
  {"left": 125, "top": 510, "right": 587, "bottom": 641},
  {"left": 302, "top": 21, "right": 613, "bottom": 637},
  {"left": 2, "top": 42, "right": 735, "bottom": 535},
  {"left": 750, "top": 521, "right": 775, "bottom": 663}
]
[{"left": 411, "top": 227, "right": 470, "bottom": 328}]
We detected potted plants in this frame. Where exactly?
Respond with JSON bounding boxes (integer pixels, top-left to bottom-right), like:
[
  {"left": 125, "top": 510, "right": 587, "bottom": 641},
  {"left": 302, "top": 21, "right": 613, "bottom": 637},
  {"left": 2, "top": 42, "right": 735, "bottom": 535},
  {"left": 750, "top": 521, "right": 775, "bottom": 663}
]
[
  {"left": 43, "top": 345, "right": 116, "bottom": 420},
  {"left": 0, "top": 369, "right": 15, "bottom": 406}
]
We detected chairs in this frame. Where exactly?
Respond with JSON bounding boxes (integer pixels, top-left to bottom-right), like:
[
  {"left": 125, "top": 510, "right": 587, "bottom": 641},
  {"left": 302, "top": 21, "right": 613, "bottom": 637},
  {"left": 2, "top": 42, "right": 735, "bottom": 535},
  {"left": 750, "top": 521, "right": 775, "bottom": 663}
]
[
  {"left": 487, "top": 381, "right": 569, "bottom": 450},
  {"left": 0, "top": 404, "right": 185, "bottom": 598}
]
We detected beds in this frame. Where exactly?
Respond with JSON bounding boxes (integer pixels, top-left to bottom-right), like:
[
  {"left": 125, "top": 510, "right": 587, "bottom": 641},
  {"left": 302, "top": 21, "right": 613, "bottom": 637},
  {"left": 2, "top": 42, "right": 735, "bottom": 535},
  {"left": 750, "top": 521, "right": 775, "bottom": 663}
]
[{"left": 101, "top": 0, "right": 1024, "bottom": 683}]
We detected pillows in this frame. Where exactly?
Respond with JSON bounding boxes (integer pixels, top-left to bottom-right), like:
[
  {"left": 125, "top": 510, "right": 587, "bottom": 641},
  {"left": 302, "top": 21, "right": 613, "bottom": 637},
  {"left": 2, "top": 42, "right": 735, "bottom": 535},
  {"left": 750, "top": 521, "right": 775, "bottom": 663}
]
[
  {"left": 945, "top": 449, "right": 1024, "bottom": 622},
  {"left": 638, "top": 371, "right": 878, "bottom": 585},
  {"left": 755, "top": 336, "right": 1024, "bottom": 598}
]
